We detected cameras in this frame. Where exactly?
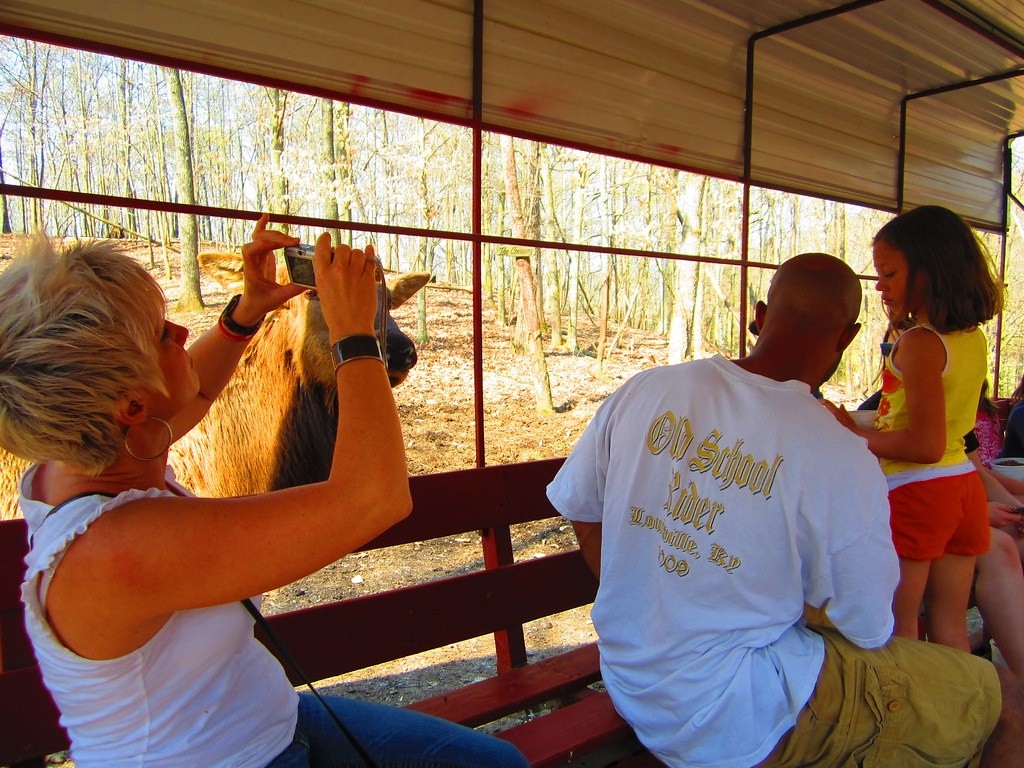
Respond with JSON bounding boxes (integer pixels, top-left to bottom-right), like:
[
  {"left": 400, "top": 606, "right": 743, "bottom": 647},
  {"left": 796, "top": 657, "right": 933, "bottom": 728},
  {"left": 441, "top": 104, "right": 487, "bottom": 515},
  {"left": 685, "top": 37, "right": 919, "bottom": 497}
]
[{"left": 283, "top": 243, "right": 334, "bottom": 290}]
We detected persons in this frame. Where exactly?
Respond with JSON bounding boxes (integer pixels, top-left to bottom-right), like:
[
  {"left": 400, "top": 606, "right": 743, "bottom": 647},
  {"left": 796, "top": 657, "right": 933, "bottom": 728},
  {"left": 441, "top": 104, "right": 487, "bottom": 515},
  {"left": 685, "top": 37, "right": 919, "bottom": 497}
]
[
  {"left": 1006, "top": 375, "right": 1024, "bottom": 457},
  {"left": 973, "top": 378, "right": 1003, "bottom": 468},
  {"left": 0, "top": 212, "right": 527, "bottom": 768},
  {"left": 856, "top": 317, "right": 1024, "bottom": 677},
  {"left": 545, "top": 253, "right": 1024, "bottom": 768},
  {"left": 817, "top": 205, "right": 1006, "bottom": 654}
]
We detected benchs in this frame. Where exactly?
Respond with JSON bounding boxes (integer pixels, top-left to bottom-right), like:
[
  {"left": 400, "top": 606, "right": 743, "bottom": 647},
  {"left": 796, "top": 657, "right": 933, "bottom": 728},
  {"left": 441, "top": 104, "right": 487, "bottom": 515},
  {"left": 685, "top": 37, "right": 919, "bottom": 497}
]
[{"left": 1, "top": 395, "right": 1024, "bottom": 766}]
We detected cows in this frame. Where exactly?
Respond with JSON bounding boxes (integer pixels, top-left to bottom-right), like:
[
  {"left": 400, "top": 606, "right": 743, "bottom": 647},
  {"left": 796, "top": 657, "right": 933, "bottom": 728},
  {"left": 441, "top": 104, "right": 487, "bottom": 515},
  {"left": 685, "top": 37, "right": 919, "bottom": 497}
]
[{"left": 0, "top": 248, "right": 432, "bottom": 539}]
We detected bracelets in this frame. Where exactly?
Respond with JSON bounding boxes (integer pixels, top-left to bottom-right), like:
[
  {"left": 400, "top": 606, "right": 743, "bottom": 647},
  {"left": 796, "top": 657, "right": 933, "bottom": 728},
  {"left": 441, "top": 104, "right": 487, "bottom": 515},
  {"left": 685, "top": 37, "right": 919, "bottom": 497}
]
[
  {"left": 219, "top": 317, "right": 260, "bottom": 342},
  {"left": 222, "top": 294, "right": 262, "bottom": 332},
  {"left": 330, "top": 335, "right": 383, "bottom": 371}
]
